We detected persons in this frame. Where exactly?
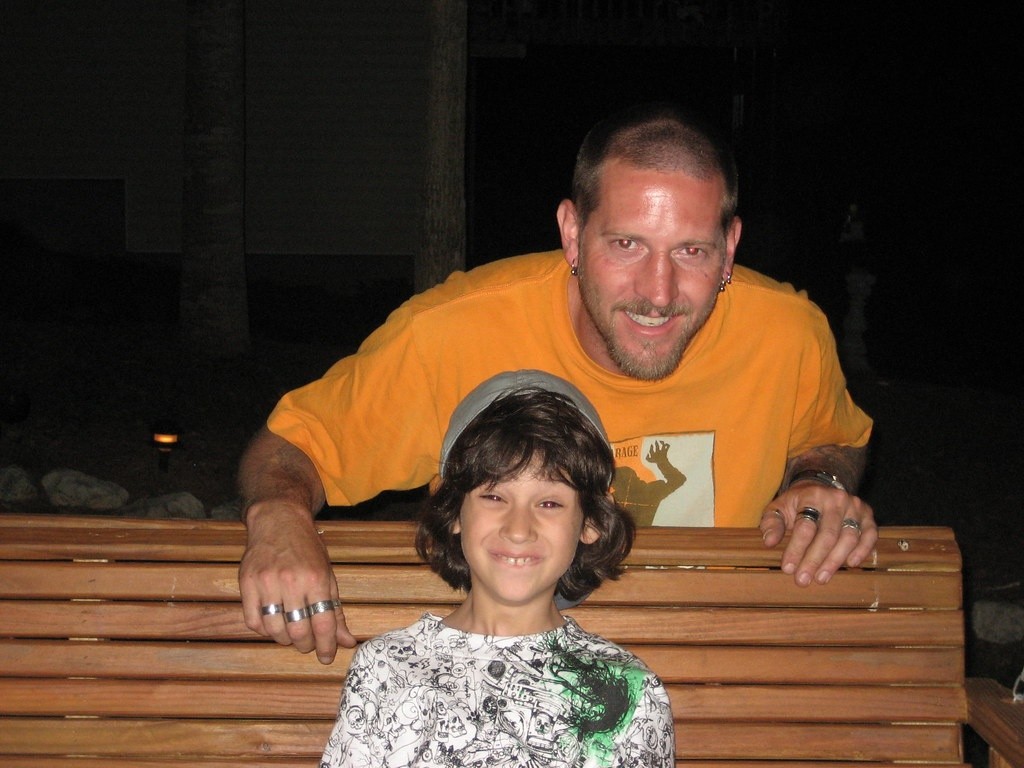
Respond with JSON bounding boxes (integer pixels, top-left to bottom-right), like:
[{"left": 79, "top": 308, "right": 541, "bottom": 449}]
[
  {"left": 235, "top": 109, "right": 879, "bottom": 666},
  {"left": 317, "top": 368, "right": 676, "bottom": 767}
]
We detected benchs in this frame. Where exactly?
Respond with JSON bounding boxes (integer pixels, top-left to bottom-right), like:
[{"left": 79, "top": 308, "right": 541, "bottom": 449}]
[{"left": 0, "top": 514, "right": 1024, "bottom": 768}]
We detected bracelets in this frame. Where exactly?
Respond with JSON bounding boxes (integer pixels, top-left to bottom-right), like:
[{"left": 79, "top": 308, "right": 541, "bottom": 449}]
[{"left": 788, "top": 471, "right": 844, "bottom": 490}]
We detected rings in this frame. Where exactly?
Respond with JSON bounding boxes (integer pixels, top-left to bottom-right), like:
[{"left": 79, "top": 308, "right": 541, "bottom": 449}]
[
  {"left": 842, "top": 519, "right": 862, "bottom": 533},
  {"left": 794, "top": 506, "right": 821, "bottom": 528},
  {"left": 764, "top": 508, "right": 784, "bottom": 519},
  {"left": 307, "top": 601, "right": 331, "bottom": 617},
  {"left": 333, "top": 600, "right": 343, "bottom": 608},
  {"left": 262, "top": 603, "right": 283, "bottom": 616},
  {"left": 284, "top": 607, "right": 309, "bottom": 624}
]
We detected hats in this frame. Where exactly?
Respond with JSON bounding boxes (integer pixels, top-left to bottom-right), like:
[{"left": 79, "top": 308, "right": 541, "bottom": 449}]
[{"left": 439, "top": 370, "right": 612, "bottom": 476}]
